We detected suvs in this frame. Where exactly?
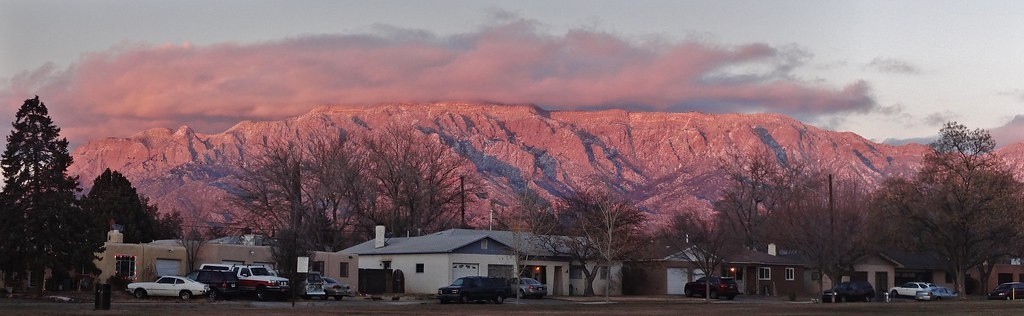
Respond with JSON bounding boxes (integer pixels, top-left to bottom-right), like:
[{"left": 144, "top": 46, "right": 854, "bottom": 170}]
[
  {"left": 184, "top": 269, "right": 240, "bottom": 302},
  {"left": 684, "top": 276, "right": 739, "bottom": 300}
]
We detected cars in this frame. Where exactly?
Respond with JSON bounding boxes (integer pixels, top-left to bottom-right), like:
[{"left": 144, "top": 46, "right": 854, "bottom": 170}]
[
  {"left": 887, "top": 282, "right": 958, "bottom": 302},
  {"left": 505, "top": 278, "right": 547, "bottom": 299},
  {"left": 125, "top": 276, "right": 210, "bottom": 303},
  {"left": 987, "top": 282, "right": 1024, "bottom": 299},
  {"left": 321, "top": 277, "right": 355, "bottom": 301},
  {"left": 821, "top": 281, "right": 875, "bottom": 302}
]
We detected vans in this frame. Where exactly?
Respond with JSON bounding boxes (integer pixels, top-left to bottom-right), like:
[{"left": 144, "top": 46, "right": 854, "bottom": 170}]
[{"left": 274, "top": 272, "right": 324, "bottom": 299}]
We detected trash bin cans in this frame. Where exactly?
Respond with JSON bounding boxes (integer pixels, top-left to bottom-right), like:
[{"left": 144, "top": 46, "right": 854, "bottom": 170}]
[{"left": 95, "top": 283, "right": 110, "bottom": 309}]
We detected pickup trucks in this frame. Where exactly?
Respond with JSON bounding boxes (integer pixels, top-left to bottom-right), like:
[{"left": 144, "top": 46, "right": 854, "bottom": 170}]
[
  {"left": 198, "top": 264, "right": 289, "bottom": 301},
  {"left": 433, "top": 276, "right": 507, "bottom": 304}
]
